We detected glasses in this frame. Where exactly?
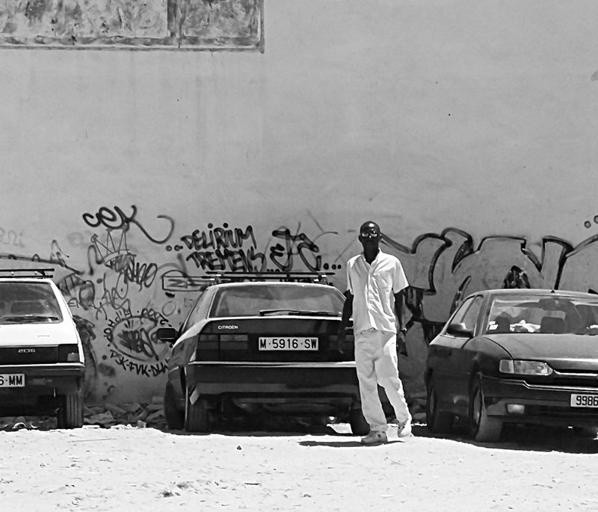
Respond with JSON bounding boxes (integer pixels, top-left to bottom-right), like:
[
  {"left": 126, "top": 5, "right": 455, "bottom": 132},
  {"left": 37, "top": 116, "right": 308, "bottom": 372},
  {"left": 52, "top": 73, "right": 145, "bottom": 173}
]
[{"left": 361, "top": 232, "right": 379, "bottom": 239}]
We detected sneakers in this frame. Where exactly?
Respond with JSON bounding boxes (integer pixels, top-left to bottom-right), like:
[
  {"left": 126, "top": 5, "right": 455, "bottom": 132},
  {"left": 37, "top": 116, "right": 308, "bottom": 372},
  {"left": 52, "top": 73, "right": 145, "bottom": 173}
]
[
  {"left": 361, "top": 431, "right": 388, "bottom": 444},
  {"left": 397, "top": 413, "right": 413, "bottom": 437}
]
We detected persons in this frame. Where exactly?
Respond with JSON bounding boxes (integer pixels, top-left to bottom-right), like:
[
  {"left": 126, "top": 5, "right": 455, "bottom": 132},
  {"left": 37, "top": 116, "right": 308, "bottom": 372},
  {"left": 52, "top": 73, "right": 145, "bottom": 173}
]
[{"left": 333, "top": 218, "right": 414, "bottom": 447}]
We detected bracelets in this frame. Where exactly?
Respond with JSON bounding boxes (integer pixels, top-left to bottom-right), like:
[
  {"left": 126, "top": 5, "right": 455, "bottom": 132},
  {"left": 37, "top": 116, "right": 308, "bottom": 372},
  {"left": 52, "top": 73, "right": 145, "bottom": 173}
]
[{"left": 399, "top": 328, "right": 408, "bottom": 334}]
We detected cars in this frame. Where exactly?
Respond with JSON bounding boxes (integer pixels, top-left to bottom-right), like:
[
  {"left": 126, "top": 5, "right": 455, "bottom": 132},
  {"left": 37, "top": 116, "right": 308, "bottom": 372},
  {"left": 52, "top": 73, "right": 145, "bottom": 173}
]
[
  {"left": 153, "top": 265, "right": 371, "bottom": 437},
  {"left": 415, "top": 286, "right": 598, "bottom": 447},
  {"left": 0, "top": 266, "right": 87, "bottom": 430}
]
